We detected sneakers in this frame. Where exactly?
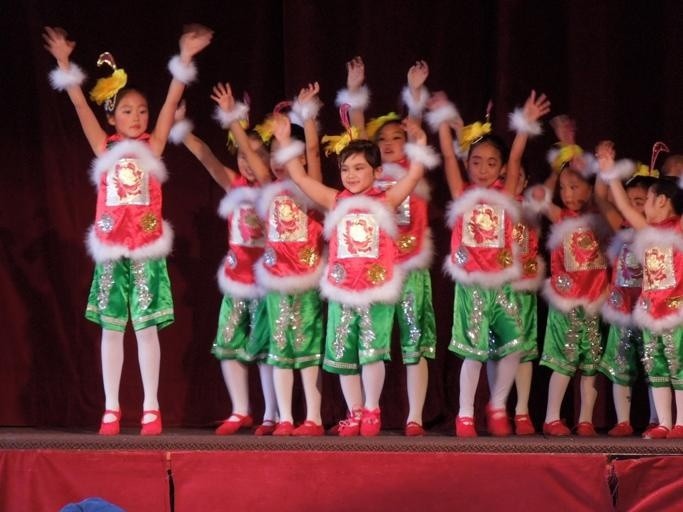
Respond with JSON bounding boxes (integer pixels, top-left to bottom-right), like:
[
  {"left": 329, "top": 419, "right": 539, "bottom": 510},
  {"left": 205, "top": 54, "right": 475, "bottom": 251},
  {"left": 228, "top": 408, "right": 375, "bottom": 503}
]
[
  {"left": 607, "top": 421, "right": 632, "bottom": 437},
  {"left": 272, "top": 420, "right": 295, "bottom": 436},
  {"left": 542, "top": 419, "right": 570, "bottom": 436},
  {"left": 666, "top": 425, "right": 682, "bottom": 438},
  {"left": 255, "top": 419, "right": 280, "bottom": 437},
  {"left": 404, "top": 422, "right": 427, "bottom": 436},
  {"left": 645, "top": 426, "right": 673, "bottom": 438},
  {"left": 329, "top": 422, "right": 338, "bottom": 434},
  {"left": 139, "top": 409, "right": 162, "bottom": 436},
  {"left": 338, "top": 409, "right": 362, "bottom": 436},
  {"left": 514, "top": 411, "right": 534, "bottom": 436},
  {"left": 360, "top": 407, "right": 381, "bottom": 438},
  {"left": 485, "top": 404, "right": 511, "bottom": 436},
  {"left": 215, "top": 413, "right": 253, "bottom": 436},
  {"left": 641, "top": 423, "right": 657, "bottom": 437},
  {"left": 292, "top": 420, "right": 325, "bottom": 436},
  {"left": 100, "top": 409, "right": 122, "bottom": 435},
  {"left": 575, "top": 420, "right": 594, "bottom": 437},
  {"left": 455, "top": 415, "right": 477, "bottom": 438}
]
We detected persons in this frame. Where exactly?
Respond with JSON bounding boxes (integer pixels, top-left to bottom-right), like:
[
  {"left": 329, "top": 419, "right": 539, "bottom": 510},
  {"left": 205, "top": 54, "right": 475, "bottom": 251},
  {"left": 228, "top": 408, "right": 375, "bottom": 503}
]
[
  {"left": 527, "top": 144, "right": 614, "bottom": 438},
  {"left": 424, "top": 89, "right": 551, "bottom": 439},
  {"left": 168, "top": 96, "right": 306, "bottom": 436},
  {"left": 593, "top": 149, "right": 683, "bottom": 440},
  {"left": 267, "top": 111, "right": 442, "bottom": 438},
  {"left": 210, "top": 81, "right": 328, "bottom": 437},
  {"left": 328, "top": 54, "right": 437, "bottom": 439},
  {"left": 596, "top": 140, "right": 659, "bottom": 441},
  {"left": 446, "top": 114, "right": 575, "bottom": 436},
  {"left": 44, "top": 24, "right": 214, "bottom": 436}
]
